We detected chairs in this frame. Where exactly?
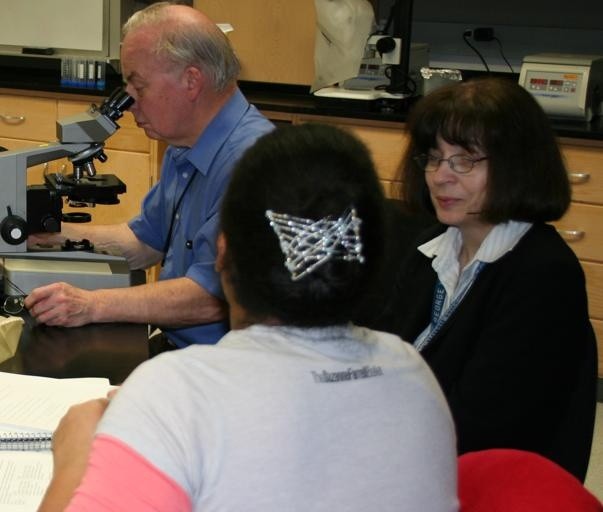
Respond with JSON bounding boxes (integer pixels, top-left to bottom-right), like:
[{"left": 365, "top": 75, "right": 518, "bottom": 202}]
[
  {"left": 454, "top": 449, "right": 603, "bottom": 512},
  {"left": 523, "top": 319, "right": 598, "bottom": 484}
]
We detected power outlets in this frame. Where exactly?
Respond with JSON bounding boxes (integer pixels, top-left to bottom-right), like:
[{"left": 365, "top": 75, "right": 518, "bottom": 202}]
[{"left": 464, "top": 28, "right": 475, "bottom": 41}]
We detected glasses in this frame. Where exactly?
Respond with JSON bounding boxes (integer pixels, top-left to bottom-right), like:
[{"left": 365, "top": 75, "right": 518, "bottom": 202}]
[
  {"left": 1, "top": 276, "right": 27, "bottom": 314},
  {"left": 409, "top": 149, "right": 486, "bottom": 174}
]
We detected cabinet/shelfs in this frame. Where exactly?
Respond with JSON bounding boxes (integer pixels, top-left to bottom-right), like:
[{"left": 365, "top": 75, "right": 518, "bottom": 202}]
[
  {"left": 0, "top": 94, "right": 169, "bottom": 286},
  {"left": 544, "top": 147, "right": 603, "bottom": 406},
  {"left": 295, "top": 113, "right": 412, "bottom": 202}
]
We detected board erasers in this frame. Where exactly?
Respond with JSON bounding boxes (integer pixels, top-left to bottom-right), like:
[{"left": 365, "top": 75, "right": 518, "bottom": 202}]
[{"left": 21, "top": 46, "right": 53, "bottom": 55}]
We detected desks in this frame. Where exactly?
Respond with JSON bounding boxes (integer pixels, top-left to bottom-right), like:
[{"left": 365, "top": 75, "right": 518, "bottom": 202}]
[{"left": 0, "top": 268, "right": 151, "bottom": 385}]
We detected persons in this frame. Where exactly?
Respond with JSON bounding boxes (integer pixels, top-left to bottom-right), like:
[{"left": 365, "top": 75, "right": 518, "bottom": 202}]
[
  {"left": 30, "top": 119, "right": 464, "bottom": 511},
  {"left": 326, "top": 73, "right": 601, "bottom": 495},
  {"left": 20, "top": 2, "right": 282, "bottom": 358}
]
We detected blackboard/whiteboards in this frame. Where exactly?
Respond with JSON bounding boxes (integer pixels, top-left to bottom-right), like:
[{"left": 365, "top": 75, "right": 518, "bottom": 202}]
[{"left": 0, "top": 0, "right": 111, "bottom": 58}]
